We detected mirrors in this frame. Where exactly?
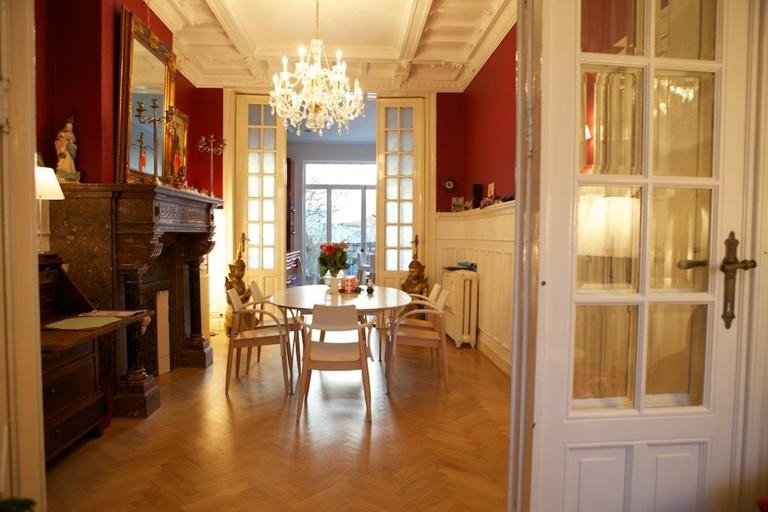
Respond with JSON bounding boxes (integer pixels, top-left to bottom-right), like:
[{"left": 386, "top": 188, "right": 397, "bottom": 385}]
[{"left": 114, "top": 2, "right": 178, "bottom": 187}]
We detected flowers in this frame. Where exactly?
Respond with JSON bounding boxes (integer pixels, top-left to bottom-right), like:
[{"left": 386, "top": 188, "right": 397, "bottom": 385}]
[{"left": 316, "top": 241, "right": 357, "bottom": 278}]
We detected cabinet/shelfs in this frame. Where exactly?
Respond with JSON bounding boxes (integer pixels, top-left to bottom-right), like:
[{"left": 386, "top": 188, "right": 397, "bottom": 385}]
[{"left": 38, "top": 253, "right": 158, "bottom": 468}]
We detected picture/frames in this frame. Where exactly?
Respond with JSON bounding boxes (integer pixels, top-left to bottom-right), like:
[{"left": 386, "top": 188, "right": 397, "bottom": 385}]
[{"left": 165, "top": 106, "right": 190, "bottom": 189}]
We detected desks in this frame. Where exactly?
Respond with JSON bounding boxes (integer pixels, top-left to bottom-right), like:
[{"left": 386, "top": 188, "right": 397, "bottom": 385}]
[{"left": 269, "top": 283, "right": 413, "bottom": 397}]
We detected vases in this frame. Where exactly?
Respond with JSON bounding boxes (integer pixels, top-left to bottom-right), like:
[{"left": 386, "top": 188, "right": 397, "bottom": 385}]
[{"left": 329, "top": 276, "right": 338, "bottom": 295}]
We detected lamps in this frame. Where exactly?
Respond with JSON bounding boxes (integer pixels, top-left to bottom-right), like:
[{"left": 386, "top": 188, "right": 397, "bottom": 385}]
[
  {"left": 33, "top": 151, "right": 66, "bottom": 255},
  {"left": 268, "top": 1, "right": 368, "bottom": 138}
]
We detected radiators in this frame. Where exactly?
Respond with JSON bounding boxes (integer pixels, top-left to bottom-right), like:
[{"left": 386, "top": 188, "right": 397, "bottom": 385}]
[{"left": 439, "top": 266, "right": 481, "bottom": 349}]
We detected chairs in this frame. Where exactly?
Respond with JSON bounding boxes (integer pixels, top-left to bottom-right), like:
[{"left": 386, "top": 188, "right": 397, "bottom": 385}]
[
  {"left": 384, "top": 281, "right": 442, "bottom": 362},
  {"left": 295, "top": 303, "right": 378, "bottom": 423},
  {"left": 224, "top": 287, "right": 294, "bottom": 397},
  {"left": 242, "top": 280, "right": 307, "bottom": 377},
  {"left": 384, "top": 287, "right": 451, "bottom": 397}
]
